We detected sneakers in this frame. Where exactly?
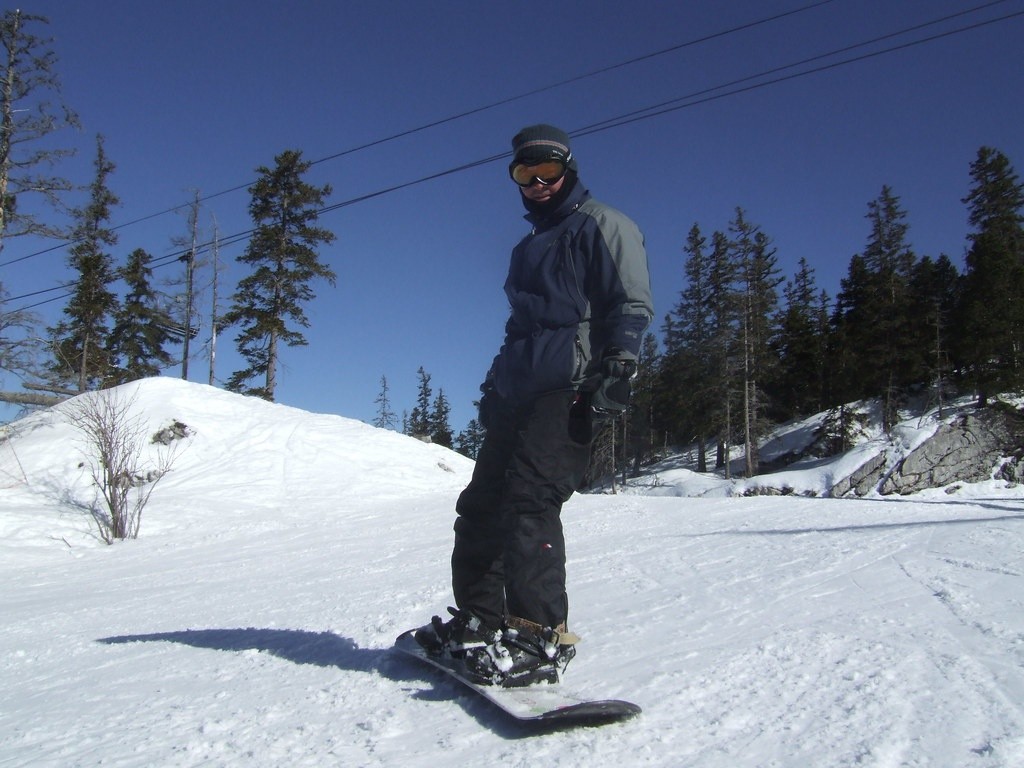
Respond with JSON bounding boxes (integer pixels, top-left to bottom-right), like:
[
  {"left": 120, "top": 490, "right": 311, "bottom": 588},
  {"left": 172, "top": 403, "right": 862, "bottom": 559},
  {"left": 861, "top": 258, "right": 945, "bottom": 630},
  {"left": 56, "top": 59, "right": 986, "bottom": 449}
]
[
  {"left": 463, "top": 626, "right": 573, "bottom": 687},
  {"left": 415, "top": 606, "right": 509, "bottom": 652}
]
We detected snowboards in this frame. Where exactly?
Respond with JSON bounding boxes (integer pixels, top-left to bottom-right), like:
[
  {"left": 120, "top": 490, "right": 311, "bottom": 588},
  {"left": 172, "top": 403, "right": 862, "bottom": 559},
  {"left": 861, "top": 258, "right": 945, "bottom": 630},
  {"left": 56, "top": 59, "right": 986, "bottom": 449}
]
[{"left": 394, "top": 627, "right": 643, "bottom": 720}]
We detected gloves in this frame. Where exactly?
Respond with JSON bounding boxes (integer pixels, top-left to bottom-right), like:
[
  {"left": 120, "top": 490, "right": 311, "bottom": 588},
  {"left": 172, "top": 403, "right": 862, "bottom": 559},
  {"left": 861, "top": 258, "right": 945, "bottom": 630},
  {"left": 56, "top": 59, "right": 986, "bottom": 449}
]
[
  {"left": 478, "top": 394, "right": 502, "bottom": 429},
  {"left": 567, "top": 358, "right": 635, "bottom": 423}
]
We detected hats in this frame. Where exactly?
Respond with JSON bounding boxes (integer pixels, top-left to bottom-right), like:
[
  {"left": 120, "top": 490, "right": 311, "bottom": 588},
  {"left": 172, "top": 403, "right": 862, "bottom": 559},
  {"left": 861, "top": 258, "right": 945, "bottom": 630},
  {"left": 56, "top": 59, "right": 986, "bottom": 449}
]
[{"left": 511, "top": 125, "right": 572, "bottom": 164}]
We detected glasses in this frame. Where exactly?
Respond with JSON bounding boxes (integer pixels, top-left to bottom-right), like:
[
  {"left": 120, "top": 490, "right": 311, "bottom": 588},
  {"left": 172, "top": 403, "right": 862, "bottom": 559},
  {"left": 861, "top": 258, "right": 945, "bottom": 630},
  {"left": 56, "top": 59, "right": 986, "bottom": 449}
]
[{"left": 508, "top": 156, "right": 565, "bottom": 188}]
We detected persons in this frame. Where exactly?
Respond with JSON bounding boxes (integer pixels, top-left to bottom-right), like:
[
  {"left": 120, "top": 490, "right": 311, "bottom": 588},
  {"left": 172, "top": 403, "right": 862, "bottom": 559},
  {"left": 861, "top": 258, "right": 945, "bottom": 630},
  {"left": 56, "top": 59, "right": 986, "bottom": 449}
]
[{"left": 414, "top": 124, "right": 654, "bottom": 689}]
